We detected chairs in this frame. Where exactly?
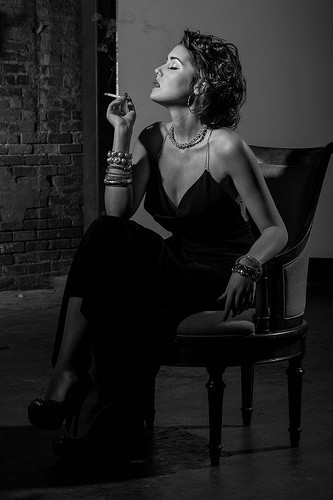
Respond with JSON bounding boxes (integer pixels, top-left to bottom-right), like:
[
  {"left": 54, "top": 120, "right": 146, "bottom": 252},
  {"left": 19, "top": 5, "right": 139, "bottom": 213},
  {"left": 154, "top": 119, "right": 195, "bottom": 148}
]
[{"left": 139, "top": 141, "right": 333, "bottom": 467}]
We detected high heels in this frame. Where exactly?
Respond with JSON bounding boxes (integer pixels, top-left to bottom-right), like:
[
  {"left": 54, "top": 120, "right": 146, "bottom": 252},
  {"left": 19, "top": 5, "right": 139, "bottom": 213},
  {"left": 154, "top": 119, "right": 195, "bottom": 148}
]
[
  {"left": 51, "top": 404, "right": 123, "bottom": 466},
  {"left": 28, "top": 370, "right": 93, "bottom": 439}
]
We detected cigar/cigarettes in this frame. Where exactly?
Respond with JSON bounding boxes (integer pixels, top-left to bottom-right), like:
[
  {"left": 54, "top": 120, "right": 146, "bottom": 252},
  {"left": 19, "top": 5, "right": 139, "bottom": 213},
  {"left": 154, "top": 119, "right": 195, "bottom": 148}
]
[{"left": 104, "top": 93, "right": 120, "bottom": 98}]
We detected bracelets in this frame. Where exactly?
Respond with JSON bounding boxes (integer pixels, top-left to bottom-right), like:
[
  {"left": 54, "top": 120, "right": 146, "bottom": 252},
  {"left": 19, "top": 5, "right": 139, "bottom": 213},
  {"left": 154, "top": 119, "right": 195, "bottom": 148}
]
[
  {"left": 104, "top": 157, "right": 133, "bottom": 186},
  {"left": 107, "top": 151, "right": 132, "bottom": 160},
  {"left": 232, "top": 255, "right": 262, "bottom": 282}
]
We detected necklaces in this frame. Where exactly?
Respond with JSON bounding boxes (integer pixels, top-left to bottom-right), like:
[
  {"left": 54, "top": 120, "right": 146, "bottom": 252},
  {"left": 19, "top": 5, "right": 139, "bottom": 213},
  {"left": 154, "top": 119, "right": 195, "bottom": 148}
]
[{"left": 170, "top": 122, "right": 208, "bottom": 149}]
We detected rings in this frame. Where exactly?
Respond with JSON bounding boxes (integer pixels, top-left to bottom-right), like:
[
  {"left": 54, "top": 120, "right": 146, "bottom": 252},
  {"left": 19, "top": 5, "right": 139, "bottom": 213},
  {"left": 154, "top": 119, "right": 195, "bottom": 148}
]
[{"left": 246, "top": 287, "right": 253, "bottom": 292}]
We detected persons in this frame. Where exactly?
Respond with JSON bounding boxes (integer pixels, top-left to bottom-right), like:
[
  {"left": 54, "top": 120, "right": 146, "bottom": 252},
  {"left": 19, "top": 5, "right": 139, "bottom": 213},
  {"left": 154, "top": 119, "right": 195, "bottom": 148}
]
[{"left": 27, "top": 30, "right": 289, "bottom": 465}]
